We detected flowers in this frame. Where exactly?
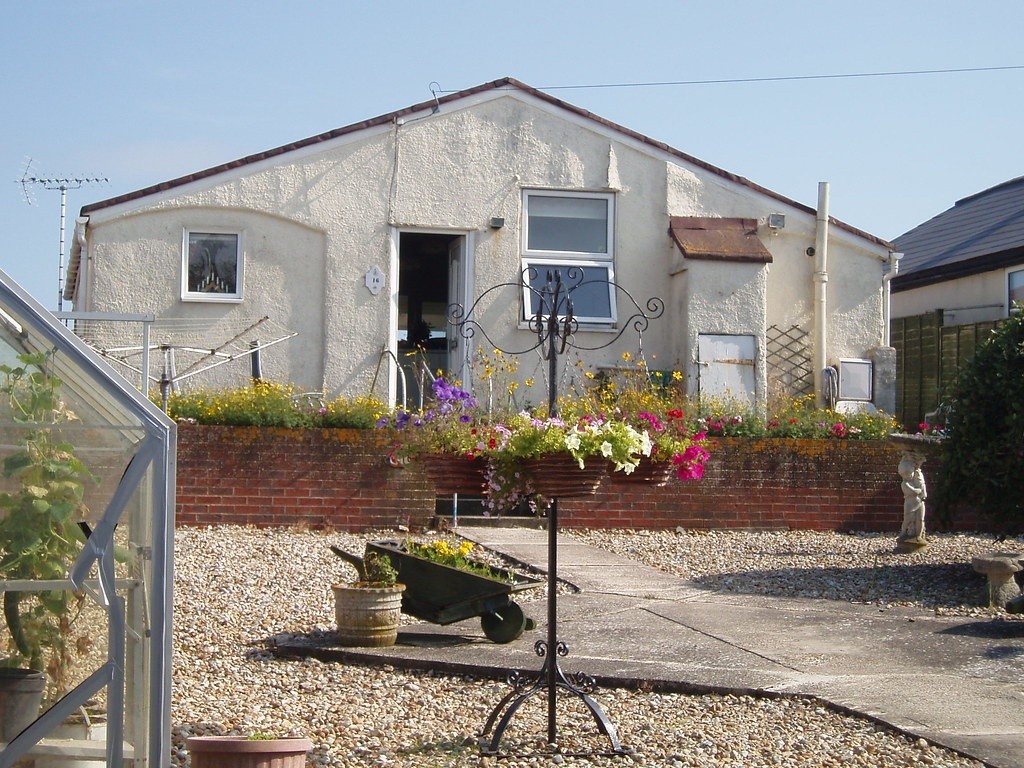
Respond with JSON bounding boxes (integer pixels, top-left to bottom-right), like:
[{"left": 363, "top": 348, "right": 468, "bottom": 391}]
[
  {"left": 377, "top": 345, "right": 718, "bottom": 519},
  {"left": 399, "top": 530, "right": 514, "bottom": 583}
]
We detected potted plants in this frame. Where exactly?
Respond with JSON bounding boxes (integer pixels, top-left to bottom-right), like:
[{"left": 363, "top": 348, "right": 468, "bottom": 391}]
[
  {"left": 187, "top": 733, "right": 312, "bottom": 768},
  {"left": 331, "top": 551, "right": 406, "bottom": 647}
]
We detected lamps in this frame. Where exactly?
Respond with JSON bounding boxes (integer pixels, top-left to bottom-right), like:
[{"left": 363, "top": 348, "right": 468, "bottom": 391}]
[{"left": 767, "top": 213, "right": 785, "bottom": 228}]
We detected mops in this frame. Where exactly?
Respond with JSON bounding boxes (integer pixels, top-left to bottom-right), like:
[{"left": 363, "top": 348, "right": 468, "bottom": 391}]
[{"left": 822, "top": 366, "right": 837, "bottom": 410}]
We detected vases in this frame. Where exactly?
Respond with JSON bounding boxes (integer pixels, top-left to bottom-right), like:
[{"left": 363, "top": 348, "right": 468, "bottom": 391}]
[
  {"left": 0, "top": 667, "right": 45, "bottom": 743},
  {"left": 518, "top": 450, "right": 609, "bottom": 498},
  {"left": 607, "top": 454, "right": 673, "bottom": 487},
  {"left": 414, "top": 452, "right": 488, "bottom": 498}
]
